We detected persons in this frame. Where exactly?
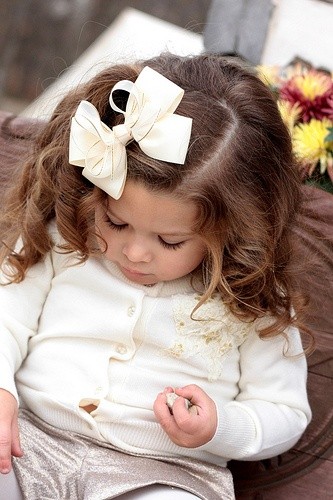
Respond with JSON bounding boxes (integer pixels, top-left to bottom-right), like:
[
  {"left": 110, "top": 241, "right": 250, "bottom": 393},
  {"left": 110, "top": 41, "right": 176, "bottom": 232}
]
[{"left": 0, "top": 52, "right": 313, "bottom": 499}]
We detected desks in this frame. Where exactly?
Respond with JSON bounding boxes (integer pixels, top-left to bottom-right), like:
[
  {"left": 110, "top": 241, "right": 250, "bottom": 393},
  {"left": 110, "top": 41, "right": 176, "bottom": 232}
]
[{"left": 0, "top": 111, "right": 332, "bottom": 500}]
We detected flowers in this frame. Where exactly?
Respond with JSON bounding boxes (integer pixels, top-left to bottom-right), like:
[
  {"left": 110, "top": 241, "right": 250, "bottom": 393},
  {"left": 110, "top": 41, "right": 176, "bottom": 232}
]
[{"left": 250, "top": 60, "right": 333, "bottom": 195}]
